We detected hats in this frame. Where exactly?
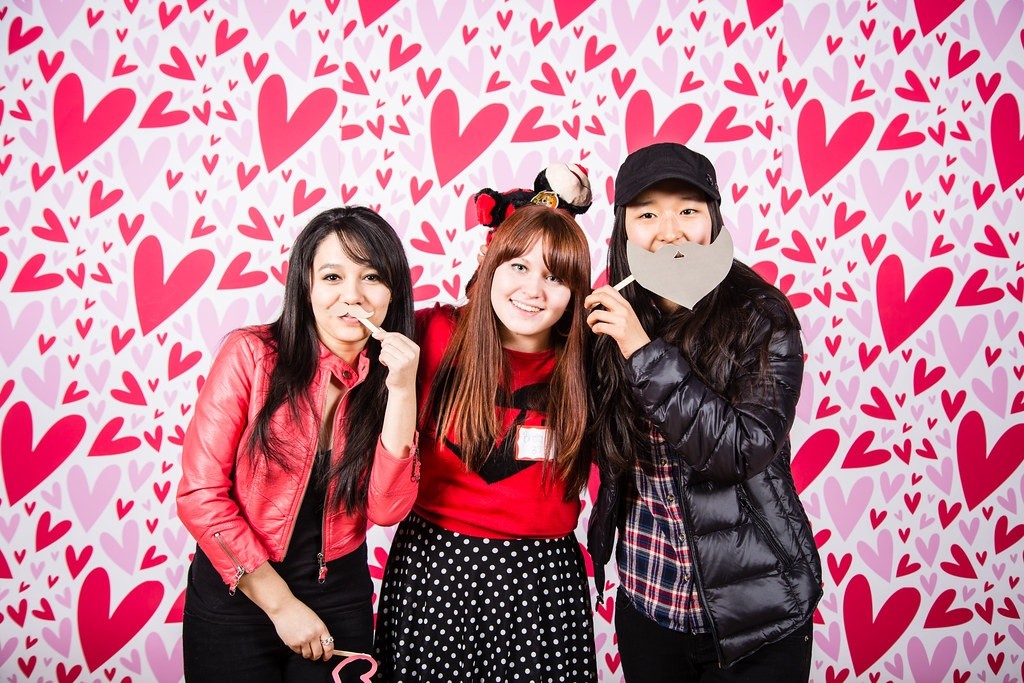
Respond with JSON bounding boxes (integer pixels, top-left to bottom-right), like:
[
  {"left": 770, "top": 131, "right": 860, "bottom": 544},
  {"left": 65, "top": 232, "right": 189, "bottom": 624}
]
[{"left": 615, "top": 143, "right": 721, "bottom": 215}]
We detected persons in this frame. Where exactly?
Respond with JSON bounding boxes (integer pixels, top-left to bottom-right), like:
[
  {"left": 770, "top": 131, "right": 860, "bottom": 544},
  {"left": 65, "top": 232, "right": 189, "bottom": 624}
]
[
  {"left": 174, "top": 204, "right": 424, "bottom": 683},
  {"left": 584, "top": 141, "right": 824, "bottom": 683},
  {"left": 374, "top": 168, "right": 599, "bottom": 683}
]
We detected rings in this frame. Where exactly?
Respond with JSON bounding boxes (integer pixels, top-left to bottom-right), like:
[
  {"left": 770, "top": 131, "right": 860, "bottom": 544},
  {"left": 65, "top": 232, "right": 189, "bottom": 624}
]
[{"left": 321, "top": 637, "right": 334, "bottom": 645}]
